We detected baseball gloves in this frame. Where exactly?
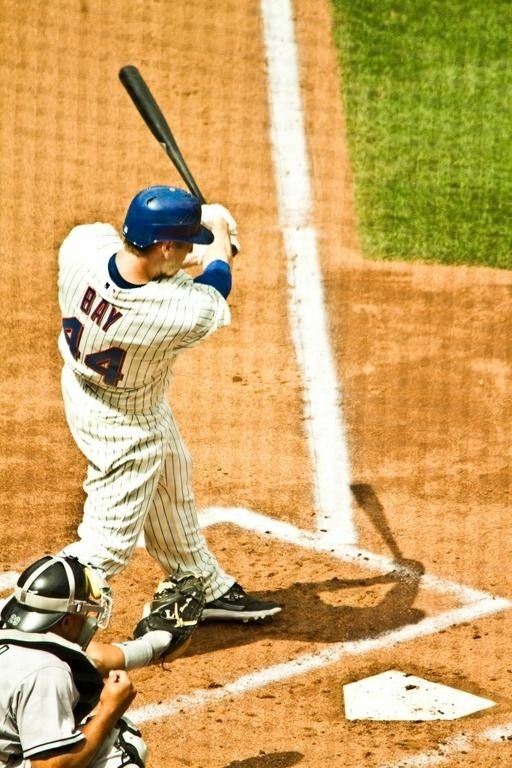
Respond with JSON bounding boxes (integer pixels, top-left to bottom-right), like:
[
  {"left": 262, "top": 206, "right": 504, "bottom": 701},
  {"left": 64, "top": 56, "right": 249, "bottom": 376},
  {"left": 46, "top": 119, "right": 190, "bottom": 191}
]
[{"left": 135, "top": 564, "right": 214, "bottom": 663}]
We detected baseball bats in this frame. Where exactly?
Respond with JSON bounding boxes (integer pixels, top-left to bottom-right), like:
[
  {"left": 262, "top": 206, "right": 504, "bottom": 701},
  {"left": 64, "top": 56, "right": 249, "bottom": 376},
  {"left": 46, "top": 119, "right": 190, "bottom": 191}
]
[{"left": 119, "top": 64, "right": 238, "bottom": 260}]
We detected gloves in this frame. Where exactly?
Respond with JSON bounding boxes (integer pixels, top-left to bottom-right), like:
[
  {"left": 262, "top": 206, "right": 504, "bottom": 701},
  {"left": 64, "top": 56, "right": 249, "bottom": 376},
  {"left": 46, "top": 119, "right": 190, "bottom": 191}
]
[
  {"left": 200, "top": 202, "right": 238, "bottom": 233},
  {"left": 198, "top": 235, "right": 240, "bottom": 265}
]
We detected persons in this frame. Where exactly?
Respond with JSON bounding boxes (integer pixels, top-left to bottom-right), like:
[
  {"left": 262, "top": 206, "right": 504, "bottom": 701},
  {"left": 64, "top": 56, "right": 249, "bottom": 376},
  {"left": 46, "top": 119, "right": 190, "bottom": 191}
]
[
  {"left": 0, "top": 553, "right": 148, "bottom": 768},
  {"left": 54, "top": 185, "right": 284, "bottom": 623},
  {"left": 88, "top": 568, "right": 206, "bottom": 677}
]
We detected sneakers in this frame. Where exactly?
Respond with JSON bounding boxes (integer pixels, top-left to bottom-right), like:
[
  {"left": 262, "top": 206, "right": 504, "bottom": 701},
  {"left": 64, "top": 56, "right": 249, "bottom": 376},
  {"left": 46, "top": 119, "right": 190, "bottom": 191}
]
[{"left": 199, "top": 582, "right": 283, "bottom": 624}]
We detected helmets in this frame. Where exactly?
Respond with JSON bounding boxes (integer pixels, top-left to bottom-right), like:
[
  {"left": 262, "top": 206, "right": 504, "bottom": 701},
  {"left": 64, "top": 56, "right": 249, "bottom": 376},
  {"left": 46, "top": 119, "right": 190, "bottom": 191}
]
[
  {"left": 0, "top": 549, "right": 113, "bottom": 651},
  {"left": 121, "top": 185, "right": 214, "bottom": 250}
]
